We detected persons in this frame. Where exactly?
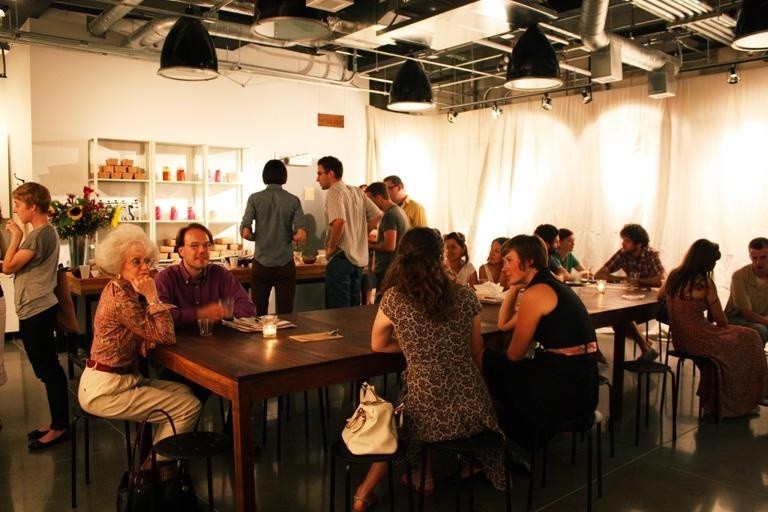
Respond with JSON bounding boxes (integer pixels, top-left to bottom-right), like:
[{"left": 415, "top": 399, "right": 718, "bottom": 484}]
[
  {"left": 479, "top": 237, "right": 510, "bottom": 291},
  {"left": 594, "top": 224, "right": 667, "bottom": 361},
  {"left": 443, "top": 232, "right": 478, "bottom": 289},
  {"left": 240, "top": 161, "right": 308, "bottom": 316},
  {"left": 656, "top": 240, "right": 768, "bottom": 419},
  {"left": 154, "top": 223, "right": 257, "bottom": 406},
  {"left": 316, "top": 156, "right": 428, "bottom": 307},
  {"left": 78, "top": 223, "right": 200, "bottom": 470},
  {"left": 533, "top": 225, "right": 611, "bottom": 364},
  {"left": 3, "top": 182, "right": 70, "bottom": 449},
  {"left": 464, "top": 234, "right": 598, "bottom": 487},
  {"left": 351, "top": 227, "right": 531, "bottom": 512},
  {"left": 724, "top": 237, "right": 768, "bottom": 348}
]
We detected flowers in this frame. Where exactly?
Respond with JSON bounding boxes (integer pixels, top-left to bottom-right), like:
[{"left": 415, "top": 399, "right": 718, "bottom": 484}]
[{"left": 46, "top": 186, "right": 124, "bottom": 256}]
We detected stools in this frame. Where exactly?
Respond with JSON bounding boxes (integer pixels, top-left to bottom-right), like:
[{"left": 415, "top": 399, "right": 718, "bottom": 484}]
[
  {"left": 626, "top": 321, "right": 705, "bottom": 452},
  {"left": 598, "top": 374, "right": 615, "bottom": 457},
  {"left": 330, "top": 412, "right": 603, "bottom": 509},
  {"left": 70, "top": 409, "right": 133, "bottom": 507},
  {"left": 152, "top": 431, "right": 227, "bottom": 509}
]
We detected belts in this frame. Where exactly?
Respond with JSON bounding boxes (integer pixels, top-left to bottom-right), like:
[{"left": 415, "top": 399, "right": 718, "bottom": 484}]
[
  {"left": 547, "top": 342, "right": 598, "bottom": 356},
  {"left": 86, "top": 359, "right": 134, "bottom": 375}
]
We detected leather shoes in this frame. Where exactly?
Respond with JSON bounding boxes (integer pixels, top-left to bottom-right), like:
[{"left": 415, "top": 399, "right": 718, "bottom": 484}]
[
  {"left": 28, "top": 430, "right": 71, "bottom": 450},
  {"left": 28, "top": 429, "right": 48, "bottom": 438}
]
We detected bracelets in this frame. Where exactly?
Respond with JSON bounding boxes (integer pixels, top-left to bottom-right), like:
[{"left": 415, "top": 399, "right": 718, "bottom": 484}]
[{"left": 147, "top": 301, "right": 163, "bottom": 305}]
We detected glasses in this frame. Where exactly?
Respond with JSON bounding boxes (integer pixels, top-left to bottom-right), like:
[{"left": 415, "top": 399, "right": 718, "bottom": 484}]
[{"left": 124, "top": 256, "right": 150, "bottom": 267}]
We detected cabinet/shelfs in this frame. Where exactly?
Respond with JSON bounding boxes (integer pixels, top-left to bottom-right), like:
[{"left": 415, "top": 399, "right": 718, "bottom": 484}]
[{"left": 84, "top": 136, "right": 245, "bottom": 262}]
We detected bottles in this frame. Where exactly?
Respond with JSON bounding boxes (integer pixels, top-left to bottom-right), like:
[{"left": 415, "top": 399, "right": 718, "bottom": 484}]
[
  {"left": 207, "top": 169, "right": 238, "bottom": 182},
  {"left": 170, "top": 206, "right": 178, "bottom": 220},
  {"left": 187, "top": 206, "right": 195, "bottom": 221},
  {"left": 176, "top": 167, "right": 185, "bottom": 181},
  {"left": 155, "top": 206, "right": 162, "bottom": 220},
  {"left": 104, "top": 199, "right": 140, "bottom": 221},
  {"left": 208, "top": 211, "right": 218, "bottom": 221},
  {"left": 162, "top": 165, "right": 171, "bottom": 181}
]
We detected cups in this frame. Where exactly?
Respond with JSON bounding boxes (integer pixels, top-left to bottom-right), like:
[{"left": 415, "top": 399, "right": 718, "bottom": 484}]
[
  {"left": 218, "top": 297, "right": 233, "bottom": 319},
  {"left": 317, "top": 250, "right": 328, "bottom": 264},
  {"left": 566, "top": 270, "right": 595, "bottom": 286},
  {"left": 263, "top": 315, "right": 278, "bottom": 337},
  {"left": 630, "top": 271, "right": 641, "bottom": 291},
  {"left": 229, "top": 256, "right": 238, "bottom": 269},
  {"left": 198, "top": 318, "right": 214, "bottom": 336},
  {"left": 293, "top": 251, "right": 301, "bottom": 265},
  {"left": 80, "top": 264, "right": 90, "bottom": 280},
  {"left": 595, "top": 279, "right": 607, "bottom": 293}
]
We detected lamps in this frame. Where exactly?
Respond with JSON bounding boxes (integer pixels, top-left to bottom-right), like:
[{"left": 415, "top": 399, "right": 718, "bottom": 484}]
[
  {"left": 441, "top": 82, "right": 601, "bottom": 124},
  {"left": 727, "top": 61, "right": 744, "bottom": 85},
  {"left": 387, "top": 56, "right": 434, "bottom": 111},
  {"left": 157, "top": 6, "right": 218, "bottom": 81},
  {"left": 732, "top": 1, "right": 768, "bottom": 52},
  {"left": 502, "top": 22, "right": 564, "bottom": 92},
  {"left": 250, "top": 0, "right": 333, "bottom": 44}
]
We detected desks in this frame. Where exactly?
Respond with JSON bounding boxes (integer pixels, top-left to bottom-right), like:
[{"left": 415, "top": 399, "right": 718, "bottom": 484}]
[
  {"left": 59, "top": 256, "right": 326, "bottom": 379},
  {"left": 144, "top": 281, "right": 660, "bottom": 511}
]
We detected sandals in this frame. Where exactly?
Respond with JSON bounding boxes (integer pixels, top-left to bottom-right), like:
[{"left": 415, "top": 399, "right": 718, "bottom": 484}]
[{"left": 401, "top": 472, "right": 434, "bottom": 497}]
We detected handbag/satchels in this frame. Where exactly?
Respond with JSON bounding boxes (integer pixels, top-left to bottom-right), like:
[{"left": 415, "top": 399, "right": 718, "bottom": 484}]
[
  {"left": 341, "top": 382, "right": 399, "bottom": 456},
  {"left": 116, "top": 469, "right": 198, "bottom": 511}
]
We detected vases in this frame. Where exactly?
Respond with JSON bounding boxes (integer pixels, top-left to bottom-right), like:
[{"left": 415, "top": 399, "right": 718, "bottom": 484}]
[{"left": 68, "top": 236, "right": 88, "bottom": 269}]
